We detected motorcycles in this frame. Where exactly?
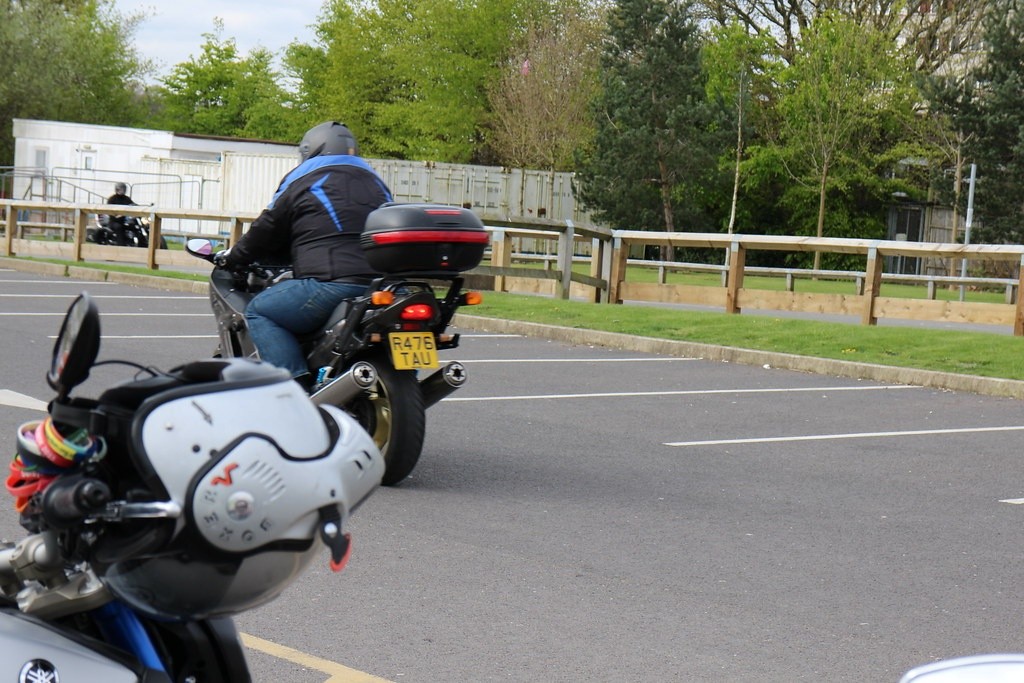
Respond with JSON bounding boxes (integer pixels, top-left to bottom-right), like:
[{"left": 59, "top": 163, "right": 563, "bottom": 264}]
[
  {"left": 0, "top": 293, "right": 386, "bottom": 683},
  {"left": 187, "top": 202, "right": 488, "bottom": 486},
  {"left": 86, "top": 203, "right": 168, "bottom": 250}
]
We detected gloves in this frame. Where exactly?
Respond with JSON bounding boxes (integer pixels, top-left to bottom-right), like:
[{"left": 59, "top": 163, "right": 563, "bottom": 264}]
[{"left": 214, "top": 246, "right": 233, "bottom": 267}]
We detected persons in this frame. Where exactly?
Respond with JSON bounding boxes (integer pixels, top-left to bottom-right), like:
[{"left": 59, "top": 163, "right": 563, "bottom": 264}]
[
  {"left": 213, "top": 121, "right": 394, "bottom": 386},
  {"left": 107, "top": 182, "right": 137, "bottom": 244}
]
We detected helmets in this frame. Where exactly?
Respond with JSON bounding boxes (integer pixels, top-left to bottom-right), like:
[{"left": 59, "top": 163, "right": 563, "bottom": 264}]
[
  {"left": 298, "top": 121, "right": 357, "bottom": 163},
  {"left": 115, "top": 182, "right": 126, "bottom": 195}
]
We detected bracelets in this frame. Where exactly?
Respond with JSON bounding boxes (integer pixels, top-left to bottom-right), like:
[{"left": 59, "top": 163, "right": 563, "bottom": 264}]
[{"left": 7, "top": 416, "right": 107, "bottom": 514}]
